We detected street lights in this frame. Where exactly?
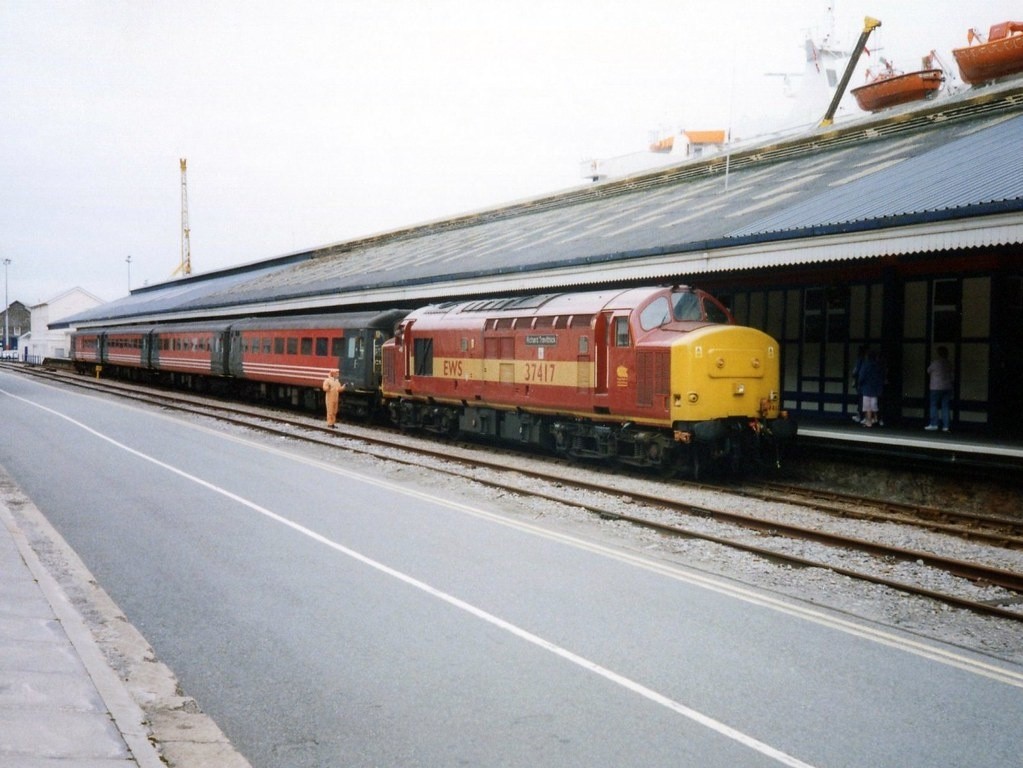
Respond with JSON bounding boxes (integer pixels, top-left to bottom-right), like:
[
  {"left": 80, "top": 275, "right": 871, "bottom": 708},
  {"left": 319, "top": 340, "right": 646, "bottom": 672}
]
[
  {"left": 2, "top": 258, "right": 12, "bottom": 350},
  {"left": 124, "top": 255, "right": 133, "bottom": 290}
]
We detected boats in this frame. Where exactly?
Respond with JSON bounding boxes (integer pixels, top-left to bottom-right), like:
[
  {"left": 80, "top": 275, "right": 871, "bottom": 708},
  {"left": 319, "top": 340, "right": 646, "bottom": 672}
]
[
  {"left": 950, "top": 20, "right": 1023, "bottom": 85},
  {"left": 850, "top": 68, "right": 944, "bottom": 112}
]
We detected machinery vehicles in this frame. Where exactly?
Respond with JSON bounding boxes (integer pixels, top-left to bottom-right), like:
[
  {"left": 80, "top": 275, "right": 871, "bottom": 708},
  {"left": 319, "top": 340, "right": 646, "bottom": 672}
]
[
  {"left": 166, "top": 156, "right": 193, "bottom": 278},
  {"left": 817, "top": 15, "right": 883, "bottom": 130}
]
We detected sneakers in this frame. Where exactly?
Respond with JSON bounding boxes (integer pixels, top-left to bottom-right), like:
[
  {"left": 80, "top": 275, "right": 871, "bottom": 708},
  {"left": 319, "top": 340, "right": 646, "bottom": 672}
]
[
  {"left": 942, "top": 428, "right": 949, "bottom": 432},
  {"left": 924, "top": 424, "right": 938, "bottom": 430}
]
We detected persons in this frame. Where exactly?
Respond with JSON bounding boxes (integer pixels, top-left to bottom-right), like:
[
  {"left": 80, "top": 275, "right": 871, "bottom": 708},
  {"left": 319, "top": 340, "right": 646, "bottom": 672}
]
[
  {"left": 924, "top": 346, "right": 953, "bottom": 430},
  {"left": 323, "top": 370, "right": 347, "bottom": 428},
  {"left": 851, "top": 347, "right": 891, "bottom": 427}
]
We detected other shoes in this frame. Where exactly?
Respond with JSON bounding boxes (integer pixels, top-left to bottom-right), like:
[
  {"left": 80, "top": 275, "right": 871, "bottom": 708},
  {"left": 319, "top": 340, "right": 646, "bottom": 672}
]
[{"left": 852, "top": 416, "right": 878, "bottom": 428}]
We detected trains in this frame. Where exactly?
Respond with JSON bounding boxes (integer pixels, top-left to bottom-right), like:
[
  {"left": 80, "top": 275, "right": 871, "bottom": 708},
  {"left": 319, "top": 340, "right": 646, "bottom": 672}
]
[{"left": 70, "top": 282, "right": 790, "bottom": 482}]
